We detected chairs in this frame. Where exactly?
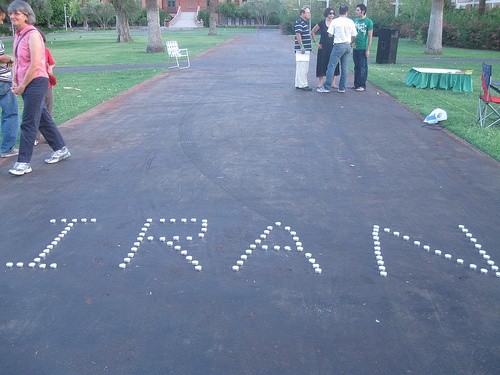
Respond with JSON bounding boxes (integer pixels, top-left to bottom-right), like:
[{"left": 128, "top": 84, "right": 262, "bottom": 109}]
[
  {"left": 166, "top": 40, "right": 190, "bottom": 70},
  {"left": 478, "top": 63, "right": 500, "bottom": 131}
]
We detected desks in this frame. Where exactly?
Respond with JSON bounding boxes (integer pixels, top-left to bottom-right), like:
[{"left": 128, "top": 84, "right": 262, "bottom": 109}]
[{"left": 404, "top": 68, "right": 473, "bottom": 92}]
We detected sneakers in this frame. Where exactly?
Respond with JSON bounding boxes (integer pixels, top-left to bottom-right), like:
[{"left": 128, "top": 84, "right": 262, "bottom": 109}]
[
  {"left": 9, "top": 162, "right": 32, "bottom": 175},
  {"left": 1, "top": 148, "right": 20, "bottom": 157},
  {"left": 338, "top": 90, "right": 345, "bottom": 93},
  {"left": 44, "top": 146, "right": 71, "bottom": 163},
  {"left": 316, "top": 87, "right": 330, "bottom": 92}
]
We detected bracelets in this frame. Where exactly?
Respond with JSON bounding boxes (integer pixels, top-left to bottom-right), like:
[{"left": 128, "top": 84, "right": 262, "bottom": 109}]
[
  {"left": 300, "top": 45, "right": 303, "bottom": 48},
  {"left": 314, "top": 40, "right": 317, "bottom": 43}
]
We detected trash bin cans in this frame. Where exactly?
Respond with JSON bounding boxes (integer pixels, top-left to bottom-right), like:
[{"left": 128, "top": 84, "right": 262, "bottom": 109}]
[{"left": 374, "top": 24, "right": 401, "bottom": 63}]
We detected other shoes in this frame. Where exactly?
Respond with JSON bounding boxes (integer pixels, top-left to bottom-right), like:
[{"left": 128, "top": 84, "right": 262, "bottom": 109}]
[
  {"left": 330, "top": 85, "right": 338, "bottom": 89},
  {"left": 350, "top": 84, "right": 356, "bottom": 88},
  {"left": 355, "top": 87, "right": 365, "bottom": 91},
  {"left": 302, "top": 86, "right": 312, "bottom": 91},
  {"left": 316, "top": 84, "right": 323, "bottom": 88}
]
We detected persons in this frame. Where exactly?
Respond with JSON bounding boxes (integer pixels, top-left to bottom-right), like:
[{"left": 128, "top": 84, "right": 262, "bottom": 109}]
[
  {"left": 347, "top": 4, "right": 373, "bottom": 92},
  {"left": 316, "top": 5, "right": 357, "bottom": 92},
  {"left": 33, "top": 30, "right": 55, "bottom": 146},
  {"left": 0, "top": 7, "right": 19, "bottom": 158},
  {"left": 7, "top": 0, "right": 71, "bottom": 176},
  {"left": 310, "top": 8, "right": 340, "bottom": 89},
  {"left": 294, "top": 7, "right": 312, "bottom": 90}
]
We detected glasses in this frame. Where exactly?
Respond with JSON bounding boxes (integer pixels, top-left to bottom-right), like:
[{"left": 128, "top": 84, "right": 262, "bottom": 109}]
[{"left": 329, "top": 12, "right": 335, "bottom": 15}]
